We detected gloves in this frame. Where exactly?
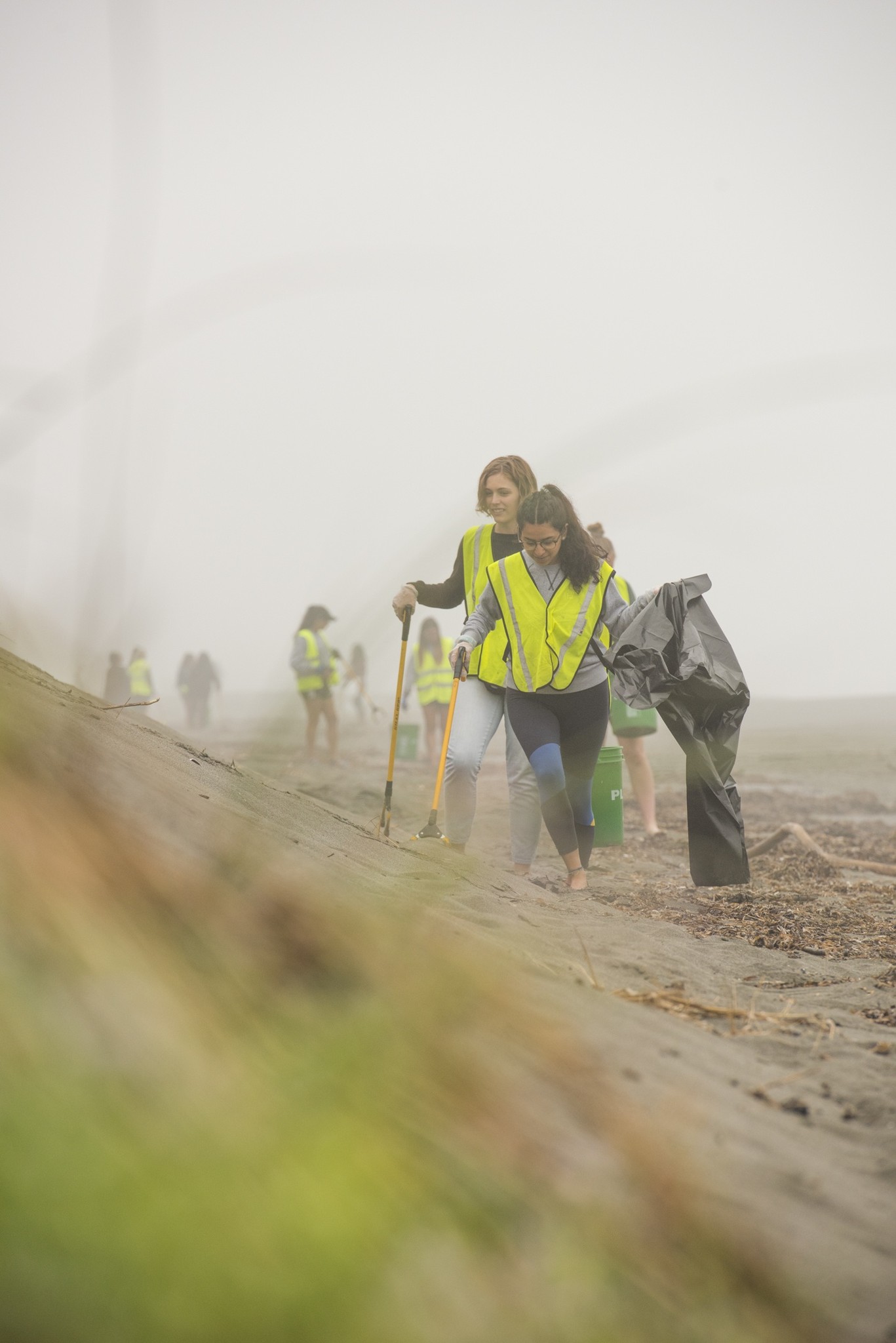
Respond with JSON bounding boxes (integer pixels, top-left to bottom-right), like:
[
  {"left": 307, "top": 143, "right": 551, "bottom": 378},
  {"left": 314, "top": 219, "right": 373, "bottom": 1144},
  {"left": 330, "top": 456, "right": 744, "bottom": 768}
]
[
  {"left": 392, "top": 584, "right": 418, "bottom": 621},
  {"left": 446, "top": 645, "right": 471, "bottom": 682}
]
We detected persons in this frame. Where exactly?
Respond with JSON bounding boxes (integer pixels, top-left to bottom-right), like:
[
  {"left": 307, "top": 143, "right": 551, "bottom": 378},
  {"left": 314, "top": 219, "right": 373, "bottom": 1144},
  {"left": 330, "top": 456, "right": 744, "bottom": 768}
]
[
  {"left": 448, "top": 483, "right": 628, "bottom": 891},
  {"left": 392, "top": 455, "right": 544, "bottom": 872},
  {"left": 344, "top": 642, "right": 369, "bottom": 723},
  {"left": 179, "top": 651, "right": 221, "bottom": 730},
  {"left": 402, "top": 618, "right": 456, "bottom": 771},
  {"left": 103, "top": 644, "right": 156, "bottom": 713},
  {"left": 291, "top": 604, "right": 348, "bottom": 768},
  {"left": 585, "top": 520, "right": 678, "bottom": 847}
]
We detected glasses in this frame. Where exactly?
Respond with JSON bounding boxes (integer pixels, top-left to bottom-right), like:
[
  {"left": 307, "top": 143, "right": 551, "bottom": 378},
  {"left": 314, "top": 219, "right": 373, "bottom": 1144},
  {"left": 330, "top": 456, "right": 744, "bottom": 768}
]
[{"left": 516, "top": 525, "right": 564, "bottom": 550}]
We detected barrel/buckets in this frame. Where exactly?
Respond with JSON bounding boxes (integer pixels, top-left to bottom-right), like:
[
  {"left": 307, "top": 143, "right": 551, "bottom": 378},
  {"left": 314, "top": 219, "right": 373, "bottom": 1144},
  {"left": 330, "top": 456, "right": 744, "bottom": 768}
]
[
  {"left": 390, "top": 720, "right": 421, "bottom": 759},
  {"left": 606, "top": 671, "right": 657, "bottom": 739},
  {"left": 591, "top": 745, "right": 623, "bottom": 849}
]
